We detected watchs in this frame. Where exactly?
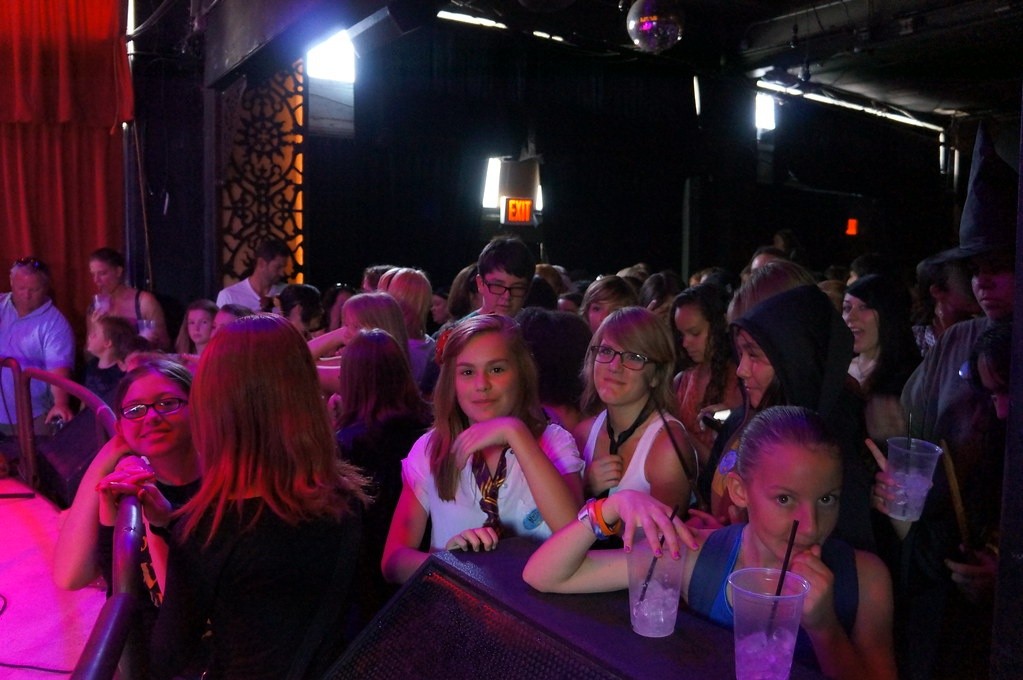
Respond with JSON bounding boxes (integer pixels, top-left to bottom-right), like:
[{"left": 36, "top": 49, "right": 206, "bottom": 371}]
[{"left": 577, "top": 504, "right": 599, "bottom": 539}]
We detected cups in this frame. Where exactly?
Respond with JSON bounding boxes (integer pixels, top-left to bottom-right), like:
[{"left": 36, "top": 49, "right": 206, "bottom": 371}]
[
  {"left": 728, "top": 568, "right": 810, "bottom": 680},
  {"left": 95, "top": 293, "right": 111, "bottom": 314},
  {"left": 623, "top": 528, "right": 686, "bottom": 638},
  {"left": 310, "top": 328, "right": 325, "bottom": 339},
  {"left": 138, "top": 319, "right": 155, "bottom": 340},
  {"left": 884, "top": 437, "right": 941, "bottom": 521}
]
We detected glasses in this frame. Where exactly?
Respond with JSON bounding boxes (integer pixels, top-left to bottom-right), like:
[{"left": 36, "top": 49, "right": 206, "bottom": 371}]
[
  {"left": 591, "top": 345, "right": 658, "bottom": 371},
  {"left": 482, "top": 275, "right": 530, "bottom": 298},
  {"left": 117, "top": 397, "right": 189, "bottom": 419},
  {"left": 14, "top": 257, "right": 46, "bottom": 274}
]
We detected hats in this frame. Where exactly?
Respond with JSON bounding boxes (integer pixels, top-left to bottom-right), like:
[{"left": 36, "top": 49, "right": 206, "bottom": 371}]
[{"left": 938, "top": 120, "right": 1019, "bottom": 258}]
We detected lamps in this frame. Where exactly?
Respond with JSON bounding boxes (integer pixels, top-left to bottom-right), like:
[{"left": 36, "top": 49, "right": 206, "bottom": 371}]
[
  {"left": 758, "top": 68, "right": 949, "bottom": 133},
  {"left": 627, "top": 1, "right": 682, "bottom": 54}
]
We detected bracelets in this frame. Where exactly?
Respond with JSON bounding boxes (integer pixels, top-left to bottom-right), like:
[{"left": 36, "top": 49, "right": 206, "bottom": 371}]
[
  {"left": 586, "top": 498, "right": 608, "bottom": 540},
  {"left": 595, "top": 498, "right": 622, "bottom": 536}
]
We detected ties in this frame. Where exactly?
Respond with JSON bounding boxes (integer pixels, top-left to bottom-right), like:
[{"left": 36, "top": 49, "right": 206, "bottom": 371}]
[
  {"left": 472, "top": 444, "right": 511, "bottom": 528},
  {"left": 599, "top": 397, "right": 656, "bottom": 500}
]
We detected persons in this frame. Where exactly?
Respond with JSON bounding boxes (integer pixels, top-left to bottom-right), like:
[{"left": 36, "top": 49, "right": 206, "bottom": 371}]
[{"left": 0, "top": 228, "right": 1017, "bottom": 680}]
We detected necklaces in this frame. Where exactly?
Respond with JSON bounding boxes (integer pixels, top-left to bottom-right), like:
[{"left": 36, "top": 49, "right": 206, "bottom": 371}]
[{"left": 857, "top": 356, "right": 877, "bottom": 379}]
